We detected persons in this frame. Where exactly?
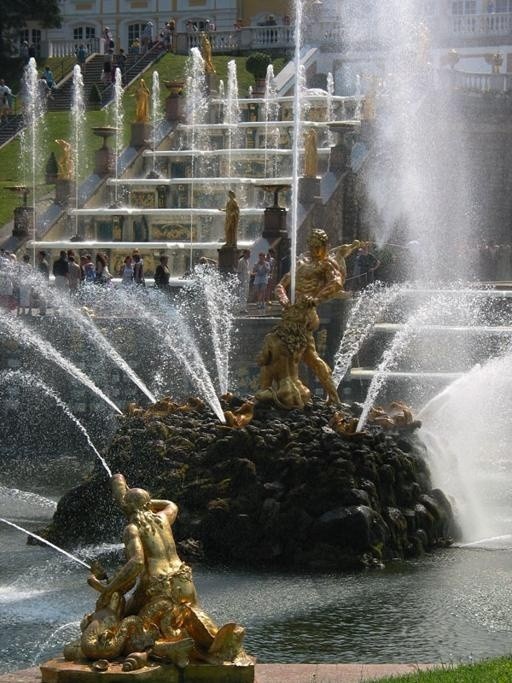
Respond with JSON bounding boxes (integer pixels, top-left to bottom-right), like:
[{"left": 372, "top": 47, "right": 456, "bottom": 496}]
[
  {"left": 0, "top": 75, "right": 12, "bottom": 124},
  {"left": 301, "top": 126, "right": 318, "bottom": 176},
  {"left": 85, "top": 484, "right": 198, "bottom": 619},
  {"left": 258, "top": 299, "right": 311, "bottom": 412},
  {"left": 55, "top": 135, "right": 74, "bottom": 180},
  {"left": 274, "top": 226, "right": 345, "bottom": 409},
  {"left": 44, "top": 63, "right": 56, "bottom": 102},
  {"left": 132, "top": 77, "right": 152, "bottom": 122},
  {"left": 219, "top": 190, "right": 239, "bottom": 246},
  {"left": 39, "top": 76, "right": 53, "bottom": 98}
]
